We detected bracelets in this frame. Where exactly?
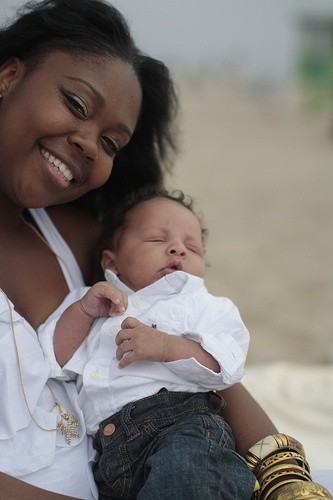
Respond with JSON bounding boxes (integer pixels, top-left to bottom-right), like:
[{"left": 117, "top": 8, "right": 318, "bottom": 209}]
[{"left": 244, "top": 433, "right": 333, "bottom": 500}]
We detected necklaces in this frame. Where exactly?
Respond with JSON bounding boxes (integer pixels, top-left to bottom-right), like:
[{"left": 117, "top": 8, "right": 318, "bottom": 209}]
[{"left": 1, "top": 287, "right": 80, "bottom": 447}]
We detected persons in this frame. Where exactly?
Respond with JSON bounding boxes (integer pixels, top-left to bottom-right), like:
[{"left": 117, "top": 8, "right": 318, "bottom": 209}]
[
  {"left": 36, "top": 190, "right": 256, "bottom": 500},
  {"left": 0, "top": 0, "right": 333, "bottom": 500}
]
